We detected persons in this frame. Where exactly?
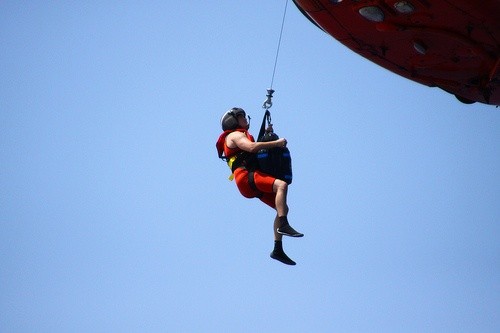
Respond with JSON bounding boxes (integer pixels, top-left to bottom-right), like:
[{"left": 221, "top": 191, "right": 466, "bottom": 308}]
[{"left": 216, "top": 107, "right": 304, "bottom": 265}]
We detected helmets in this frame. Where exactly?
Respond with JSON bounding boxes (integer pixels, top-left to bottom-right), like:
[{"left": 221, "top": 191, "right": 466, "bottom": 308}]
[{"left": 221, "top": 106, "right": 246, "bottom": 131}]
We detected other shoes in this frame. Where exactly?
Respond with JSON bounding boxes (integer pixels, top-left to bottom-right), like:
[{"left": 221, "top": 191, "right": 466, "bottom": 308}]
[
  {"left": 277, "top": 223, "right": 305, "bottom": 237},
  {"left": 270, "top": 248, "right": 296, "bottom": 265}
]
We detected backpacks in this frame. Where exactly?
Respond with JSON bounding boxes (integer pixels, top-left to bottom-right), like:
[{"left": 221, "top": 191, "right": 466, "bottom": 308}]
[{"left": 256, "top": 132, "right": 292, "bottom": 185}]
